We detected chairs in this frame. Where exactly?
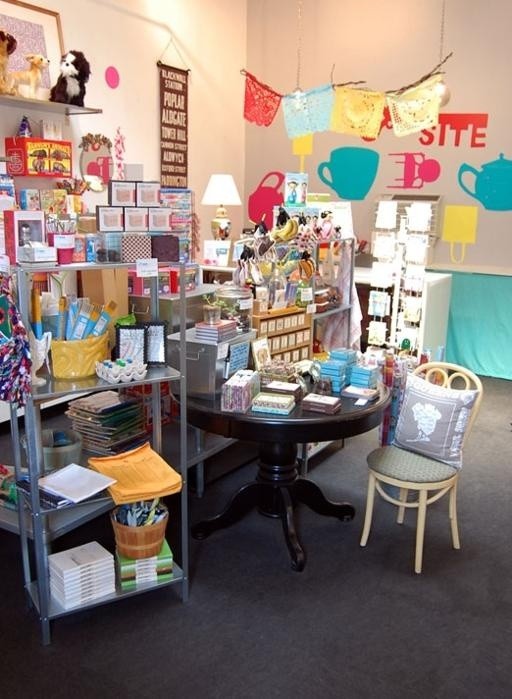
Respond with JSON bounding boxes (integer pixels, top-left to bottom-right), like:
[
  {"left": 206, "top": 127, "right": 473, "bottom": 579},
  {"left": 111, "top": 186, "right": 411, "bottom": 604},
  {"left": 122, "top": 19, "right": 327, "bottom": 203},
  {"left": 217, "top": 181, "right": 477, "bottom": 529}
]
[{"left": 359, "top": 360, "right": 483, "bottom": 575}]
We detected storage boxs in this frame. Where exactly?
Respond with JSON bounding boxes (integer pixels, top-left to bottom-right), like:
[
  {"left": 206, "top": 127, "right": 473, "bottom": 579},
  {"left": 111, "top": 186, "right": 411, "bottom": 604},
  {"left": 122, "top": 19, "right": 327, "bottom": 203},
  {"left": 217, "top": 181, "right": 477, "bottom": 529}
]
[
  {"left": 165, "top": 326, "right": 259, "bottom": 401},
  {"left": 259, "top": 366, "right": 302, "bottom": 388},
  {"left": 50, "top": 328, "right": 110, "bottom": 379},
  {"left": 5, "top": 136, "right": 72, "bottom": 177}
]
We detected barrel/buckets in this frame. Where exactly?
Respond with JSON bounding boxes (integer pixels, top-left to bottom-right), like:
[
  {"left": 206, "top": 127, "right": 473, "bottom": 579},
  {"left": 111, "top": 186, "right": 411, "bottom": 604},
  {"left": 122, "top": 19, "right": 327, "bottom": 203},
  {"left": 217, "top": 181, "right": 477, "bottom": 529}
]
[
  {"left": 19, "top": 426, "right": 82, "bottom": 474},
  {"left": 110, "top": 501, "right": 169, "bottom": 559}
]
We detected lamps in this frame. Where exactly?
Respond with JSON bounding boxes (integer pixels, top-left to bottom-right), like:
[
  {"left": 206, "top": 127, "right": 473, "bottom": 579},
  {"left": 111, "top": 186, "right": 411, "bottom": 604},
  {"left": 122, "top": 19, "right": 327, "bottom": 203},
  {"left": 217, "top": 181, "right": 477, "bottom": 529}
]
[{"left": 199, "top": 173, "right": 243, "bottom": 240}]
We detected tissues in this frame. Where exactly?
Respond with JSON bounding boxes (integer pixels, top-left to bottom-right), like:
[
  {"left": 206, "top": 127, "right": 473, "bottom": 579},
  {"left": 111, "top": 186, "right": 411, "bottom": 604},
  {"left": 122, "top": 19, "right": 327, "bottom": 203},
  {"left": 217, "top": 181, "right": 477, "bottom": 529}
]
[{"left": 19, "top": 240, "right": 59, "bottom": 262}]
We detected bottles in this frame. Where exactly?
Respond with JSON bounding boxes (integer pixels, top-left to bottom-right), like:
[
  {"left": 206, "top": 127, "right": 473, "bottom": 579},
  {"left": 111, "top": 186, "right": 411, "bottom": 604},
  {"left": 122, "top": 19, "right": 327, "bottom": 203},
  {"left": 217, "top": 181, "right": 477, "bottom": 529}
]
[
  {"left": 94, "top": 228, "right": 123, "bottom": 263},
  {"left": 268, "top": 268, "right": 283, "bottom": 307},
  {"left": 15, "top": 113, "right": 34, "bottom": 138}
]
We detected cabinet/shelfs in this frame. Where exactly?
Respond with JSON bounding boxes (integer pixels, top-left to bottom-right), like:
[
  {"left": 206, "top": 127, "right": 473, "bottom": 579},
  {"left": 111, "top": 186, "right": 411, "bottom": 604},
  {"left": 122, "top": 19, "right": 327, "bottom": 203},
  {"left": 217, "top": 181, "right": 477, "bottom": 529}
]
[
  {"left": 8, "top": 259, "right": 189, "bottom": 646},
  {"left": 252, "top": 306, "right": 310, "bottom": 364},
  {"left": 128, "top": 281, "right": 223, "bottom": 336},
  {"left": 0, "top": 92, "right": 102, "bottom": 426}
]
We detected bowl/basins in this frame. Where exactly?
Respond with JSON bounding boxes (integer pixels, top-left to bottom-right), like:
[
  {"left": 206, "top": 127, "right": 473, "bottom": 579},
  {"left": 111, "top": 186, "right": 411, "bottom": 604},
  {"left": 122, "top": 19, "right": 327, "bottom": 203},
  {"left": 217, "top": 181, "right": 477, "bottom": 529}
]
[{"left": 19, "top": 430, "right": 83, "bottom": 472}]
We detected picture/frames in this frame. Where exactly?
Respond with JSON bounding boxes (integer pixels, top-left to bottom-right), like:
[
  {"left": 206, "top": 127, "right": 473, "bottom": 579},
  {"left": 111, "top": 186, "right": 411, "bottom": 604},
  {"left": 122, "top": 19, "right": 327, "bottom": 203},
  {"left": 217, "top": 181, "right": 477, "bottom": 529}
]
[
  {"left": 96, "top": 179, "right": 171, "bottom": 233},
  {"left": 0, "top": 0, "right": 65, "bottom": 102}
]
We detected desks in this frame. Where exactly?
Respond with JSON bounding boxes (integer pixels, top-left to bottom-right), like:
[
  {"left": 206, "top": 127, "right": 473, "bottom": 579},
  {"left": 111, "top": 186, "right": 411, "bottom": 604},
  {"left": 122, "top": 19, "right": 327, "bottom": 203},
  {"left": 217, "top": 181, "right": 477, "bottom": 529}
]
[
  {"left": 175, "top": 366, "right": 393, "bottom": 572},
  {"left": 199, "top": 264, "right": 453, "bottom": 362}
]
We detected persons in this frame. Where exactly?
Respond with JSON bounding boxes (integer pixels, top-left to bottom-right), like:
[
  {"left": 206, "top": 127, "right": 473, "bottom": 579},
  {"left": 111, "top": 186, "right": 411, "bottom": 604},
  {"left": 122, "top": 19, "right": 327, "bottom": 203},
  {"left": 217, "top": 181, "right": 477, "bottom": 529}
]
[{"left": 18, "top": 223, "right": 32, "bottom": 246}]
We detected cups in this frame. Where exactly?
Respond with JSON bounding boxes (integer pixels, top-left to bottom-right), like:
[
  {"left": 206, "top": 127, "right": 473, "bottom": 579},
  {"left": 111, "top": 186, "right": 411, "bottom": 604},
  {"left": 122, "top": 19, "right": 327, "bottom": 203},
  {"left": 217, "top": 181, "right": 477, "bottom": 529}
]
[
  {"left": 312, "top": 375, "right": 333, "bottom": 396},
  {"left": 316, "top": 146, "right": 380, "bottom": 201}
]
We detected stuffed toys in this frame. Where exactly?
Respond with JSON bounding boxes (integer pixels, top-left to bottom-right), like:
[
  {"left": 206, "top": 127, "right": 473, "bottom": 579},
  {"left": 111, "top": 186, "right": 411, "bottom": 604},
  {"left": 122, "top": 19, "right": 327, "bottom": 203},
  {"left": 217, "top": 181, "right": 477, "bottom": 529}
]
[
  {"left": 50, "top": 51, "right": 92, "bottom": 109},
  {"left": 7, "top": 53, "right": 50, "bottom": 98}
]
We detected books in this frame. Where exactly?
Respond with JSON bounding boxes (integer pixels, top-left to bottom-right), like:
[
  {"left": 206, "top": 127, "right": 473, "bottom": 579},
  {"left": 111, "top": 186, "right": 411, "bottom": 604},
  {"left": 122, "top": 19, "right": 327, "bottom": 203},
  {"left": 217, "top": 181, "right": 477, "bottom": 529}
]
[
  {"left": 44, "top": 541, "right": 115, "bottom": 611},
  {"left": 12, "top": 465, "right": 117, "bottom": 511},
  {"left": 195, "top": 319, "right": 239, "bottom": 344},
  {"left": 65, "top": 390, "right": 151, "bottom": 457}
]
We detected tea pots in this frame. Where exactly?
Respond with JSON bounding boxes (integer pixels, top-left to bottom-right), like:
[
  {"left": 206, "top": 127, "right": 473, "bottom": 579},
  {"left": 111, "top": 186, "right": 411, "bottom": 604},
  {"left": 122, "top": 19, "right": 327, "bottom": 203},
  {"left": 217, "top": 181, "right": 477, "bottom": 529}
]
[{"left": 458, "top": 152, "right": 511, "bottom": 212}]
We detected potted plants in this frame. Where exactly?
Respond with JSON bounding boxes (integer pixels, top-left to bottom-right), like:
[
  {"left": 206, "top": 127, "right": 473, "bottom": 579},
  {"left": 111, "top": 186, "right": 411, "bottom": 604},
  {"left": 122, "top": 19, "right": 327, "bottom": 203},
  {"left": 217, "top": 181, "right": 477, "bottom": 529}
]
[{"left": 201, "top": 293, "right": 228, "bottom": 325}]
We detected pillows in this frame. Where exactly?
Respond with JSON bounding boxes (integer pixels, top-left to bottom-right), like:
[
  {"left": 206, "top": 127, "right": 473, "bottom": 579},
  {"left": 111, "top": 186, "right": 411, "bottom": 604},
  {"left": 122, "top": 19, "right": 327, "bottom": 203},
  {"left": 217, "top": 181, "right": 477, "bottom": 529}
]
[{"left": 391, "top": 372, "right": 480, "bottom": 471}]
